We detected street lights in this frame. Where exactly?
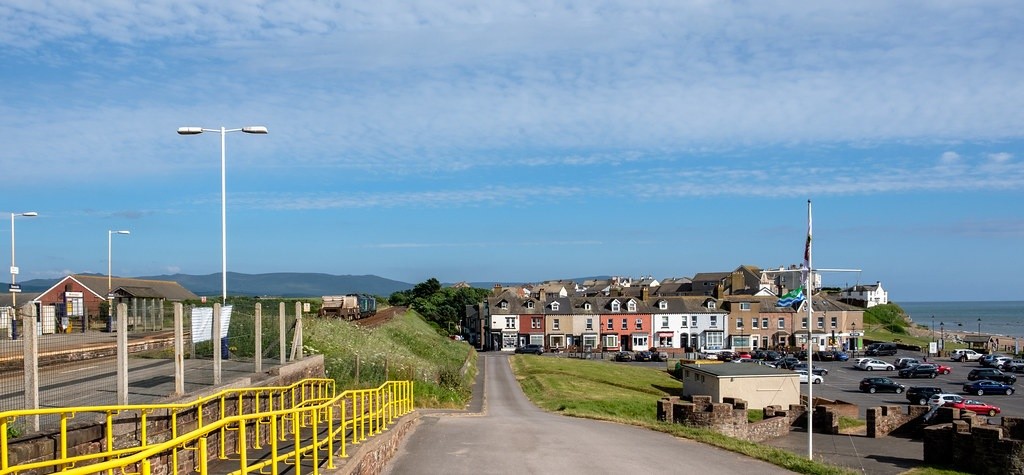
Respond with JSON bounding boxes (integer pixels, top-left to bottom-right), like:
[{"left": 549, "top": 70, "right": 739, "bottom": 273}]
[
  {"left": 449, "top": 321, "right": 454, "bottom": 331},
  {"left": 740, "top": 324, "right": 744, "bottom": 353},
  {"left": 931, "top": 316, "right": 935, "bottom": 342},
  {"left": 939, "top": 321, "right": 944, "bottom": 338},
  {"left": 851, "top": 321, "right": 856, "bottom": 358},
  {"left": 460, "top": 319, "right": 463, "bottom": 335},
  {"left": 177, "top": 125, "right": 269, "bottom": 361},
  {"left": 11, "top": 211, "right": 38, "bottom": 340},
  {"left": 977, "top": 318, "right": 981, "bottom": 337},
  {"left": 600, "top": 322, "right": 604, "bottom": 360},
  {"left": 106, "top": 229, "right": 131, "bottom": 333}
]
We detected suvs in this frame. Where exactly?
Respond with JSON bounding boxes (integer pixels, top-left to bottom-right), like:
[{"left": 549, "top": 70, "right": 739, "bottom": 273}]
[
  {"left": 927, "top": 394, "right": 963, "bottom": 407},
  {"left": 950, "top": 348, "right": 983, "bottom": 362},
  {"left": 979, "top": 354, "right": 1024, "bottom": 373},
  {"left": 898, "top": 363, "right": 939, "bottom": 379},
  {"left": 906, "top": 387, "right": 944, "bottom": 406},
  {"left": 515, "top": 344, "right": 544, "bottom": 355},
  {"left": 965, "top": 368, "right": 1017, "bottom": 385},
  {"left": 859, "top": 377, "right": 906, "bottom": 394}
]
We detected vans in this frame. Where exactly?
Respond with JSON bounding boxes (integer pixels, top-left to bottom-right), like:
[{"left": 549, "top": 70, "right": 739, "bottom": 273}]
[{"left": 865, "top": 342, "right": 898, "bottom": 357}]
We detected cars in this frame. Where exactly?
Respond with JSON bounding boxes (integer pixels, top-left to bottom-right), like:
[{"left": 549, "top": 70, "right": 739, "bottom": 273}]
[
  {"left": 790, "top": 363, "right": 828, "bottom": 376},
  {"left": 616, "top": 351, "right": 632, "bottom": 362},
  {"left": 812, "top": 351, "right": 850, "bottom": 362},
  {"left": 853, "top": 358, "right": 874, "bottom": 368},
  {"left": 699, "top": 348, "right": 801, "bottom": 370},
  {"left": 963, "top": 380, "right": 1015, "bottom": 396},
  {"left": 860, "top": 359, "right": 895, "bottom": 372},
  {"left": 635, "top": 351, "right": 652, "bottom": 362},
  {"left": 793, "top": 370, "right": 824, "bottom": 385},
  {"left": 893, "top": 357, "right": 951, "bottom": 375},
  {"left": 944, "top": 398, "right": 1001, "bottom": 417},
  {"left": 653, "top": 351, "right": 668, "bottom": 362}
]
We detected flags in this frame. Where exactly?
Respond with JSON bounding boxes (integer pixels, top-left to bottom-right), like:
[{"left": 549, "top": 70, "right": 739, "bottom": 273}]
[{"left": 803, "top": 235, "right": 810, "bottom": 284}]
